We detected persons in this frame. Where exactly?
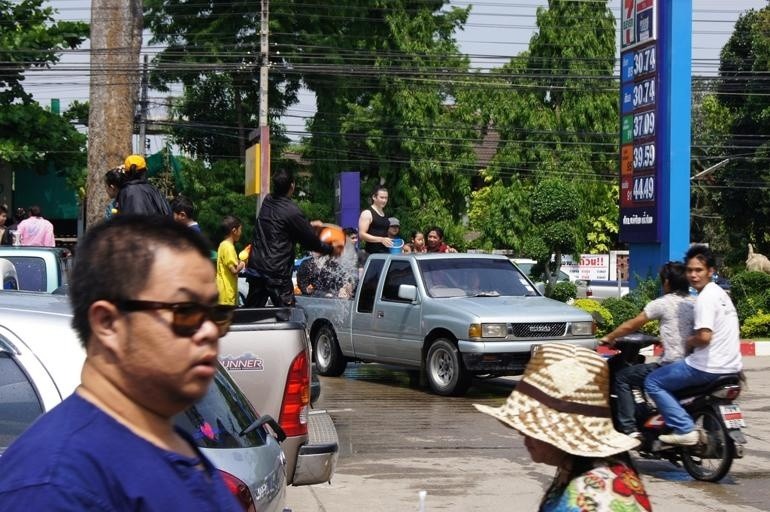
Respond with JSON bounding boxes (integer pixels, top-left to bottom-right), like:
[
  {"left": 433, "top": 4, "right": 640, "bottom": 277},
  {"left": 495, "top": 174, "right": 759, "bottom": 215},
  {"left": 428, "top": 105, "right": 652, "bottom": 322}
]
[
  {"left": 599, "top": 260, "right": 696, "bottom": 444},
  {"left": 294, "top": 183, "right": 459, "bottom": 300},
  {"left": 170, "top": 194, "right": 201, "bottom": 233},
  {"left": 643, "top": 244, "right": 743, "bottom": 446},
  {"left": 99, "top": 153, "right": 170, "bottom": 217},
  {"left": 472, "top": 341, "right": 653, "bottom": 512},
  {"left": 1, "top": 205, "right": 56, "bottom": 248},
  {"left": 240, "top": 166, "right": 344, "bottom": 309},
  {"left": 1, "top": 211, "right": 245, "bottom": 511},
  {"left": 214, "top": 215, "right": 251, "bottom": 305}
]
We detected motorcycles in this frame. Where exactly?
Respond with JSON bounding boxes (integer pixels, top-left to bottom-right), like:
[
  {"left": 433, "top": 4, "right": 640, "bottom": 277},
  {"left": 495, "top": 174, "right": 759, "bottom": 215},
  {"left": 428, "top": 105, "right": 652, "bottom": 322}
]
[{"left": 601, "top": 331, "right": 747, "bottom": 481}]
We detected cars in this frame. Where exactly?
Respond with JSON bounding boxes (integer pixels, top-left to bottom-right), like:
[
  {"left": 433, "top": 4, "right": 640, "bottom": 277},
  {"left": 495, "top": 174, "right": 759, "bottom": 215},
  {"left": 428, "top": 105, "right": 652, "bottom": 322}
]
[
  {"left": 0, "top": 288, "right": 286, "bottom": 510},
  {"left": 509, "top": 259, "right": 592, "bottom": 299}
]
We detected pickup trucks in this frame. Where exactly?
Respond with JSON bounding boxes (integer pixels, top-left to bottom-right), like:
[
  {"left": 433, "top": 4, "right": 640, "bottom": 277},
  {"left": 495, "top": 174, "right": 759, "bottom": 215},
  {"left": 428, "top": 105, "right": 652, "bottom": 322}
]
[
  {"left": 0, "top": 258, "right": 340, "bottom": 486},
  {"left": 290, "top": 253, "right": 597, "bottom": 395}
]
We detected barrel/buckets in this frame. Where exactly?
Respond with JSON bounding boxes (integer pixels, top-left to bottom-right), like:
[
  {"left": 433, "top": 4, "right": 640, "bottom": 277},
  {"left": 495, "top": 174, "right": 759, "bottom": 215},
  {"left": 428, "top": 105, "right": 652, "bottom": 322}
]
[{"left": 389, "top": 239, "right": 404, "bottom": 254}]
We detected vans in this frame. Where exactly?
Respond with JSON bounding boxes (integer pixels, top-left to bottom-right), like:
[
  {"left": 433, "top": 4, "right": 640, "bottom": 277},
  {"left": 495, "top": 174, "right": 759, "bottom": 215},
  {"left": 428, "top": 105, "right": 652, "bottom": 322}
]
[{"left": 0, "top": 246, "right": 70, "bottom": 294}]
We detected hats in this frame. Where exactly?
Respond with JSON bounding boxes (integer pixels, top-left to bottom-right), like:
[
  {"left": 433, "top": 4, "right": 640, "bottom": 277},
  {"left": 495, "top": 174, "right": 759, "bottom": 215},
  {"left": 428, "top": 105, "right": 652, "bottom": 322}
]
[
  {"left": 125, "top": 155, "right": 150, "bottom": 172},
  {"left": 388, "top": 217, "right": 400, "bottom": 227},
  {"left": 471, "top": 341, "right": 642, "bottom": 458}
]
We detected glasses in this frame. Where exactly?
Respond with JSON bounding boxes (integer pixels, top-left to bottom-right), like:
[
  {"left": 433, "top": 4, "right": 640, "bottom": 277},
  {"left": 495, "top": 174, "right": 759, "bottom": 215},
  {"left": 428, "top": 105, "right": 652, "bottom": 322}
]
[{"left": 74, "top": 302, "right": 240, "bottom": 337}]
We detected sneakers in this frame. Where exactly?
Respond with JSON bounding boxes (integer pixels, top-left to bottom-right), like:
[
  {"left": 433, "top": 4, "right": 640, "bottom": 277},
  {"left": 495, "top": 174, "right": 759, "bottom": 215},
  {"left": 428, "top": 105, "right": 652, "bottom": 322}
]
[
  {"left": 626, "top": 431, "right": 644, "bottom": 440},
  {"left": 657, "top": 430, "right": 700, "bottom": 446}
]
